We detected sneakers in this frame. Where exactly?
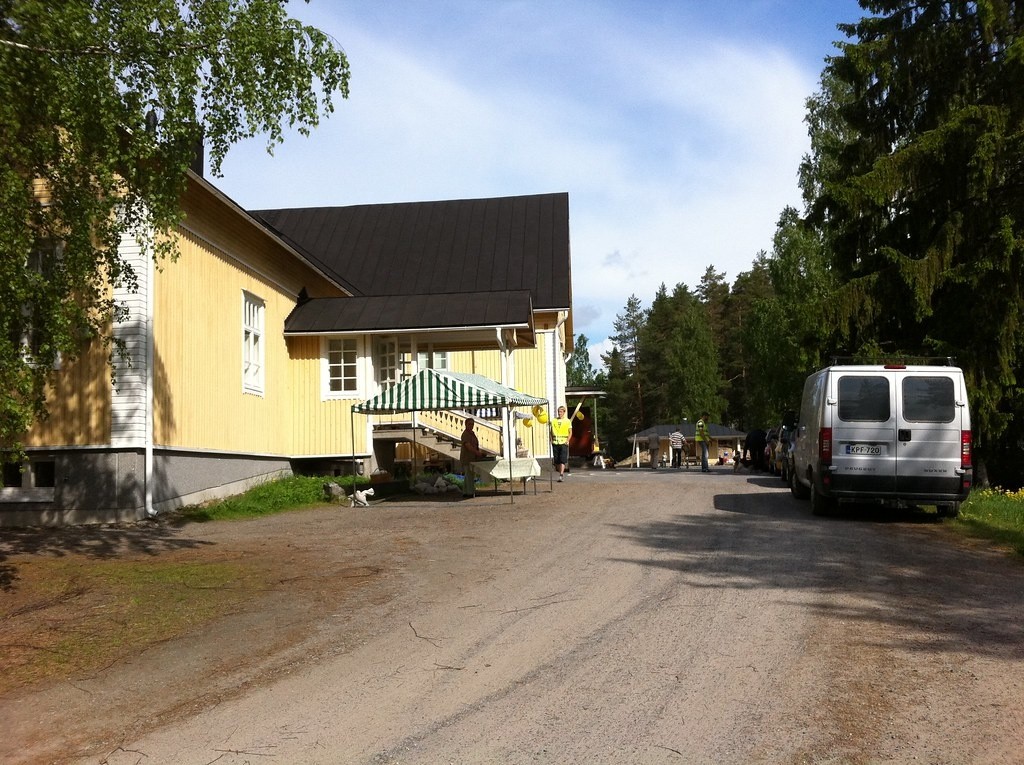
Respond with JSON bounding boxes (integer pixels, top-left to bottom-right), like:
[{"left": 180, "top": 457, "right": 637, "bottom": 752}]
[
  {"left": 702, "top": 469, "right": 712, "bottom": 473},
  {"left": 558, "top": 476, "right": 564, "bottom": 483}
]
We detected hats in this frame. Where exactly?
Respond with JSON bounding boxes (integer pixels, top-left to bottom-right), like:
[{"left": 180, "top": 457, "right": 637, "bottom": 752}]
[
  {"left": 650, "top": 428, "right": 657, "bottom": 432},
  {"left": 675, "top": 427, "right": 680, "bottom": 430}
]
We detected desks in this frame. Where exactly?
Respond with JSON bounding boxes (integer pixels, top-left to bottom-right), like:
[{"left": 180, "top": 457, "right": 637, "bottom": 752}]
[{"left": 470, "top": 457, "right": 543, "bottom": 498}]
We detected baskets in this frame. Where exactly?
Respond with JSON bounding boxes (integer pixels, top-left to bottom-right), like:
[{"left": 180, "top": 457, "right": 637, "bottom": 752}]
[{"left": 366, "top": 467, "right": 392, "bottom": 482}]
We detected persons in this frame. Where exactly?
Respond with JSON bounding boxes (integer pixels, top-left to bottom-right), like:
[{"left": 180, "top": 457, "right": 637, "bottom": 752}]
[
  {"left": 460, "top": 418, "right": 483, "bottom": 498},
  {"left": 648, "top": 429, "right": 661, "bottom": 470},
  {"left": 695, "top": 411, "right": 710, "bottom": 472},
  {"left": 550, "top": 407, "right": 572, "bottom": 482},
  {"left": 668, "top": 428, "right": 690, "bottom": 468}
]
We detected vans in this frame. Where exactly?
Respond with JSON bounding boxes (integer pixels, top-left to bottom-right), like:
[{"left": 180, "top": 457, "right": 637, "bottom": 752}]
[{"left": 792, "top": 364, "right": 973, "bottom": 518}]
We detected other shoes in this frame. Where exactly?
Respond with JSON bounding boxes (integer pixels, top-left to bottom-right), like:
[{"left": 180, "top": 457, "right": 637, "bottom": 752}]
[
  {"left": 652, "top": 467, "right": 656, "bottom": 470},
  {"left": 670, "top": 465, "right": 677, "bottom": 468},
  {"left": 678, "top": 466, "right": 682, "bottom": 469},
  {"left": 464, "top": 493, "right": 475, "bottom": 499},
  {"left": 473, "top": 493, "right": 480, "bottom": 497}
]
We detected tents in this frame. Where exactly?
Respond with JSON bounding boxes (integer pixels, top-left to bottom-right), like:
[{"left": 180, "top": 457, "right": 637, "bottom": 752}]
[{"left": 351, "top": 368, "right": 552, "bottom": 504}]
